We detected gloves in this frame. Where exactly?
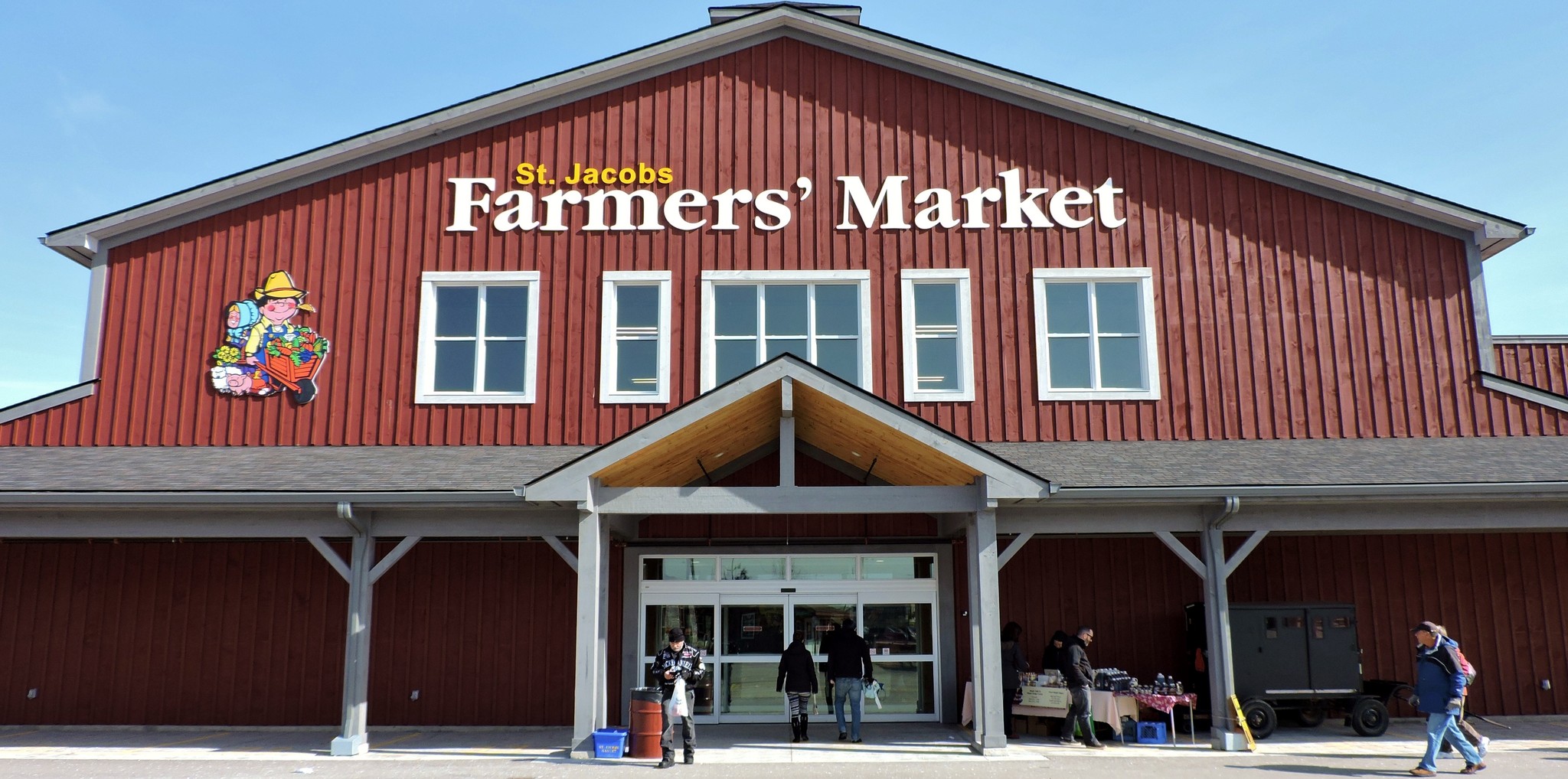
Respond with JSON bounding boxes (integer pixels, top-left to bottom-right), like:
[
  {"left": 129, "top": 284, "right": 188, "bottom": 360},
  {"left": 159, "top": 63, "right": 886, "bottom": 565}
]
[
  {"left": 1445, "top": 698, "right": 1461, "bottom": 712},
  {"left": 681, "top": 669, "right": 690, "bottom": 680},
  {"left": 1408, "top": 695, "right": 1420, "bottom": 708}
]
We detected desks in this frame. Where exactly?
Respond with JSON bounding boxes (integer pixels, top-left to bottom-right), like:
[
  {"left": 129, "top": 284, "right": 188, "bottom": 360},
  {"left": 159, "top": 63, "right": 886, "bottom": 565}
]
[
  {"left": 961, "top": 681, "right": 1140, "bottom": 745},
  {"left": 1115, "top": 691, "right": 1197, "bottom": 749}
]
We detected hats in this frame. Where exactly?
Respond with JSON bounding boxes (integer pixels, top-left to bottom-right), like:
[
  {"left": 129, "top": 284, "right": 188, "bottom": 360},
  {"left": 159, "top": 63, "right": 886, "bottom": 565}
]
[{"left": 668, "top": 627, "right": 684, "bottom": 642}]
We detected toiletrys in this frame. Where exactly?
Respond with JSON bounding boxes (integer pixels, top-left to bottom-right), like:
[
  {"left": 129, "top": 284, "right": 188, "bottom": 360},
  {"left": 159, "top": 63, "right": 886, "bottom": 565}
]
[{"left": 1019, "top": 672, "right": 1036, "bottom": 686}]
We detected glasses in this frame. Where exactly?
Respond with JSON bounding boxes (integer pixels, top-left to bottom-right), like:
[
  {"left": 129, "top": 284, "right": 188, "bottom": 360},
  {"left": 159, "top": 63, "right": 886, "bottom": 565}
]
[{"left": 1086, "top": 633, "right": 1093, "bottom": 640}]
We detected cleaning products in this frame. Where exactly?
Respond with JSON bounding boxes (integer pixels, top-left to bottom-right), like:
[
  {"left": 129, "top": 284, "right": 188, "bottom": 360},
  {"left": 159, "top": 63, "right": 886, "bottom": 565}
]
[{"left": 1095, "top": 667, "right": 1131, "bottom": 692}]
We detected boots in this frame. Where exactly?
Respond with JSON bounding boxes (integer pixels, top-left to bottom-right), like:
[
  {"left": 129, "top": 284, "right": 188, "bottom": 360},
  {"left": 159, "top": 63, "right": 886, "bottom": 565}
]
[
  {"left": 791, "top": 717, "right": 801, "bottom": 743},
  {"left": 800, "top": 714, "right": 809, "bottom": 741}
]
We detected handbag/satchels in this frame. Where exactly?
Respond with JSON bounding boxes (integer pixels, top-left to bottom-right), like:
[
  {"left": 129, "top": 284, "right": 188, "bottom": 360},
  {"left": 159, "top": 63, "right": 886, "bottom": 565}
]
[
  {"left": 812, "top": 694, "right": 818, "bottom": 715},
  {"left": 862, "top": 679, "right": 882, "bottom": 709},
  {"left": 668, "top": 675, "right": 688, "bottom": 717}
]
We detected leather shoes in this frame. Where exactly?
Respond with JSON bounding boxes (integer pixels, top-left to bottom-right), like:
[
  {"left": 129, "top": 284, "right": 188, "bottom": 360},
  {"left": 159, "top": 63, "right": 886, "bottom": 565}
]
[
  {"left": 684, "top": 756, "right": 693, "bottom": 765},
  {"left": 659, "top": 759, "right": 674, "bottom": 768}
]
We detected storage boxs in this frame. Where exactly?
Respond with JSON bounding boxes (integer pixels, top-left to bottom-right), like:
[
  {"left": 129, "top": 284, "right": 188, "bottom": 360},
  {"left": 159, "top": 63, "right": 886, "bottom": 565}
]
[
  {"left": 1114, "top": 722, "right": 1137, "bottom": 743},
  {"left": 592, "top": 728, "right": 629, "bottom": 758},
  {"left": 1137, "top": 721, "right": 1167, "bottom": 744}
]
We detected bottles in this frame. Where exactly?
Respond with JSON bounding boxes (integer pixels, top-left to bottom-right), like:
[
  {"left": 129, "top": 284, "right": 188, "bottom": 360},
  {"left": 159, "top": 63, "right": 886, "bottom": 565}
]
[
  {"left": 1103, "top": 669, "right": 1108, "bottom": 674},
  {"left": 1108, "top": 668, "right": 1112, "bottom": 672},
  {"left": 1155, "top": 681, "right": 1161, "bottom": 690},
  {"left": 1157, "top": 673, "right": 1165, "bottom": 684},
  {"left": 1103, "top": 672, "right": 1116, "bottom": 692},
  {"left": 1162, "top": 683, "right": 1168, "bottom": 691},
  {"left": 1123, "top": 671, "right": 1130, "bottom": 690},
  {"left": 1116, "top": 671, "right": 1125, "bottom": 690},
  {"left": 1176, "top": 681, "right": 1183, "bottom": 696},
  {"left": 1168, "top": 684, "right": 1175, "bottom": 691},
  {"left": 1112, "top": 668, "right": 1120, "bottom": 672},
  {"left": 1094, "top": 669, "right": 1103, "bottom": 691},
  {"left": 1114, "top": 672, "right": 1119, "bottom": 690},
  {"left": 1167, "top": 676, "right": 1174, "bottom": 684},
  {"left": 1120, "top": 671, "right": 1128, "bottom": 690}
]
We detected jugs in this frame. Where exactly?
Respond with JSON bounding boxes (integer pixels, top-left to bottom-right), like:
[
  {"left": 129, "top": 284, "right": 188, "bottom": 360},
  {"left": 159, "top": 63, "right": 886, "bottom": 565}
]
[
  {"left": 1130, "top": 678, "right": 1138, "bottom": 687},
  {"left": 1130, "top": 685, "right": 1154, "bottom": 694}
]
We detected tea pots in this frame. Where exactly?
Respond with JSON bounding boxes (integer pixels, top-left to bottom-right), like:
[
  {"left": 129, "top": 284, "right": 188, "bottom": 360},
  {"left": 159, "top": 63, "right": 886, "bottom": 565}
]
[{"left": 1092, "top": 669, "right": 1100, "bottom": 688}]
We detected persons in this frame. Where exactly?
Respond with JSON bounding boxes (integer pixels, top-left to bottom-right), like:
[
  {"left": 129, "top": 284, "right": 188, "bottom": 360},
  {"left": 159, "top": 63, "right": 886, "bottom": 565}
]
[
  {"left": 1040, "top": 630, "right": 1067, "bottom": 675},
  {"left": 1059, "top": 626, "right": 1108, "bottom": 750},
  {"left": 1436, "top": 625, "right": 1490, "bottom": 759},
  {"left": 650, "top": 627, "right": 706, "bottom": 768},
  {"left": 1408, "top": 621, "right": 1486, "bottom": 777},
  {"left": 828, "top": 620, "right": 874, "bottom": 743},
  {"left": 1001, "top": 622, "right": 1030, "bottom": 741},
  {"left": 775, "top": 630, "right": 819, "bottom": 742}
]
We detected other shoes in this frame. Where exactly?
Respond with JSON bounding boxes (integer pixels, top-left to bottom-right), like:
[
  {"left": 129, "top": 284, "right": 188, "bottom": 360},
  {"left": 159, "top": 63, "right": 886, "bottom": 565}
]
[
  {"left": 1086, "top": 742, "right": 1107, "bottom": 750},
  {"left": 1006, "top": 734, "right": 1019, "bottom": 739},
  {"left": 1409, "top": 766, "right": 1437, "bottom": 777},
  {"left": 1436, "top": 751, "right": 1454, "bottom": 760},
  {"left": 1059, "top": 737, "right": 1082, "bottom": 746},
  {"left": 852, "top": 738, "right": 861, "bottom": 743},
  {"left": 839, "top": 733, "right": 847, "bottom": 740},
  {"left": 1460, "top": 762, "right": 1486, "bottom": 774},
  {"left": 1477, "top": 737, "right": 1490, "bottom": 758}
]
